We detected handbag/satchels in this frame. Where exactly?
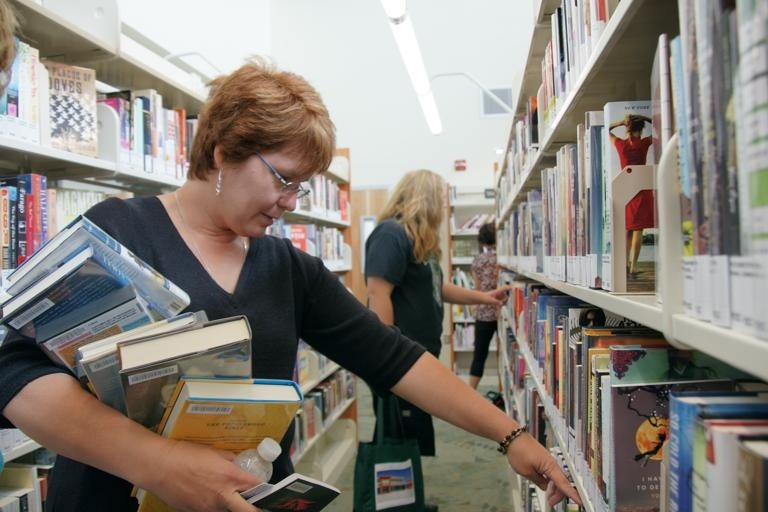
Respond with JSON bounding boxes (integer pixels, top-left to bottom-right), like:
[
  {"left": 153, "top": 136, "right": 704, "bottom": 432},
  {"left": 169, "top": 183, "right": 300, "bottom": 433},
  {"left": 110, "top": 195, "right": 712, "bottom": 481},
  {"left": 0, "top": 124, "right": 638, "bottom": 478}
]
[{"left": 354, "top": 441, "right": 422, "bottom": 511}]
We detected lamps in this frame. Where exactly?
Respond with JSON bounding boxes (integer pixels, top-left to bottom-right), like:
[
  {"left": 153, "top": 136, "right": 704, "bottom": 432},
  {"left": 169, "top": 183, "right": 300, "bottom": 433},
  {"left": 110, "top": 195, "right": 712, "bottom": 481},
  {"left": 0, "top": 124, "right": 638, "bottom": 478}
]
[{"left": 384, "top": 0, "right": 445, "bottom": 138}]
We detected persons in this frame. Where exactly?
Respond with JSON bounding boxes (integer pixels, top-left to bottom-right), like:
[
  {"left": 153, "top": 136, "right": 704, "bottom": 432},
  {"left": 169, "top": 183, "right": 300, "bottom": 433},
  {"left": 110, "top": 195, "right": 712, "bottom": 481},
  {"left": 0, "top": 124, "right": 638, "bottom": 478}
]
[
  {"left": 0, "top": 0, "right": 26, "bottom": 96},
  {"left": 364, "top": 169, "right": 512, "bottom": 512},
  {"left": 468, "top": 222, "right": 503, "bottom": 390},
  {"left": 1, "top": 55, "right": 583, "bottom": 512}
]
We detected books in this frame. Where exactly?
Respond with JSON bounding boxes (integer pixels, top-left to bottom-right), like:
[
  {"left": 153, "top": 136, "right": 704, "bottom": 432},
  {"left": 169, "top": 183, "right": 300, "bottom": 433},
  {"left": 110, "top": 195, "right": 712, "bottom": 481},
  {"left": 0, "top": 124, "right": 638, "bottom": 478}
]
[
  {"left": 0, "top": 215, "right": 342, "bottom": 511},
  {"left": 339, "top": 369, "right": 354, "bottom": 400},
  {"left": 448, "top": 205, "right": 504, "bottom": 376},
  {"left": 496, "top": 101, "right": 657, "bottom": 296},
  {"left": 265, "top": 174, "right": 348, "bottom": 260},
  {"left": 2, "top": 174, "right": 134, "bottom": 270},
  {"left": 652, "top": 0, "right": 768, "bottom": 256},
  {"left": 499, "top": 269, "right": 768, "bottom": 512},
  {"left": 2, "top": 42, "right": 199, "bottom": 180}
]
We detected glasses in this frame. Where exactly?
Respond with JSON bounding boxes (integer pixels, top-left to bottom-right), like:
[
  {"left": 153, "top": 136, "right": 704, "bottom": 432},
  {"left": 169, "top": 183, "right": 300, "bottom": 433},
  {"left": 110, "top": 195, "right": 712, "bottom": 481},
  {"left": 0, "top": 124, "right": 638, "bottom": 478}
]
[{"left": 253, "top": 150, "right": 310, "bottom": 199}]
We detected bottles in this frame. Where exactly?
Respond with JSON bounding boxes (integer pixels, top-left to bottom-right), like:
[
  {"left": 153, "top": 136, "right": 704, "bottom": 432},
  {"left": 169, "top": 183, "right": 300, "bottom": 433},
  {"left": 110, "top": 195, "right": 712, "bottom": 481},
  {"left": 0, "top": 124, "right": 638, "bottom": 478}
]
[{"left": 233, "top": 437, "right": 282, "bottom": 482}]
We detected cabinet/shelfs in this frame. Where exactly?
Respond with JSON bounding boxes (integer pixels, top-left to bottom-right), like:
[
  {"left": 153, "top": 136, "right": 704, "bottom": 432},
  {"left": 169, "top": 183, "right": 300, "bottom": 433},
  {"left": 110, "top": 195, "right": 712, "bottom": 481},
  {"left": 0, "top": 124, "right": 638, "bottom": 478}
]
[
  {"left": 446, "top": 196, "right": 494, "bottom": 362},
  {"left": 436, "top": 0, "right": 768, "bottom": 512},
  {"left": 1, "top": 0, "right": 356, "bottom": 512}
]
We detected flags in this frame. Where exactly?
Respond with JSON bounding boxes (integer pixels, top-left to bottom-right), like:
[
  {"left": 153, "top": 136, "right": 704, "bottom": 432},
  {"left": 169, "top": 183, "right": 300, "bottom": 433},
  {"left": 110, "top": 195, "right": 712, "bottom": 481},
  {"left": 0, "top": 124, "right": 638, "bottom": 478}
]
[{"left": 493, "top": 0, "right": 627, "bottom": 204}]
